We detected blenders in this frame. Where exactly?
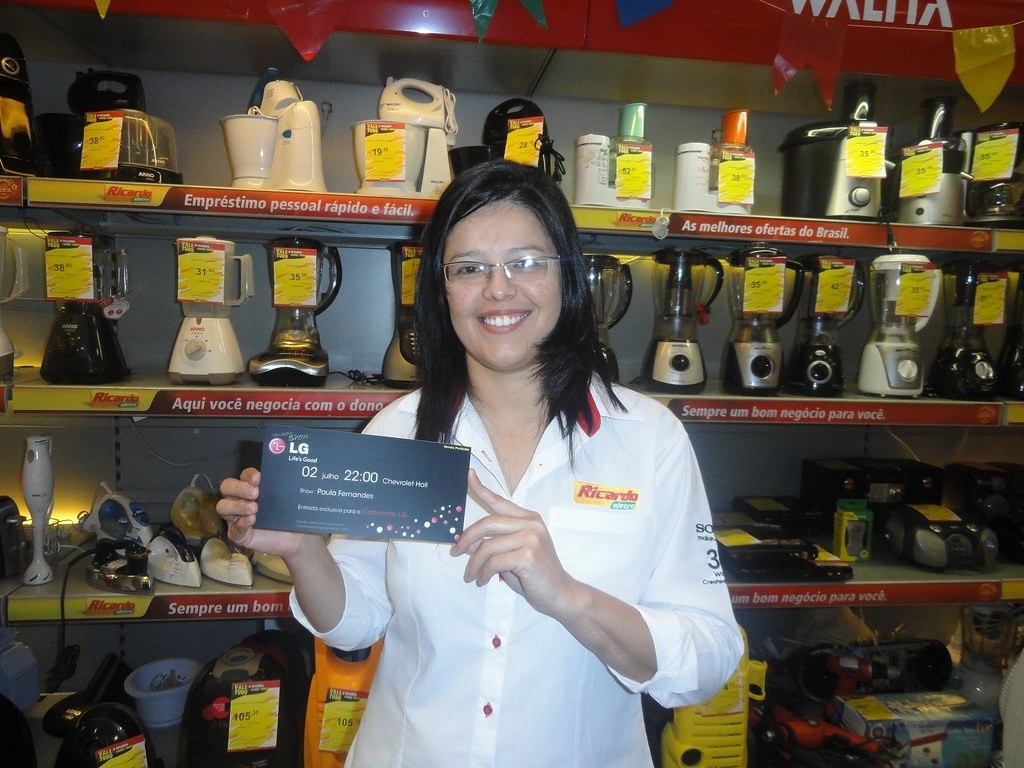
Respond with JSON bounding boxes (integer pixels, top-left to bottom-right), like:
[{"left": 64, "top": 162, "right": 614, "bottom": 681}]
[{"left": 0, "top": 10, "right": 1023, "bottom": 768}]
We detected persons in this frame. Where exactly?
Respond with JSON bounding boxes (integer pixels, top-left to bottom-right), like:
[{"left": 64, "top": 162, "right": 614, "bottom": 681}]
[{"left": 214, "top": 155, "right": 744, "bottom": 767}]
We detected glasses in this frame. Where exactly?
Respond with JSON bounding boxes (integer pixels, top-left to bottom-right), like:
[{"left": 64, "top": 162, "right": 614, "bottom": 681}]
[{"left": 439, "top": 257, "right": 561, "bottom": 282}]
